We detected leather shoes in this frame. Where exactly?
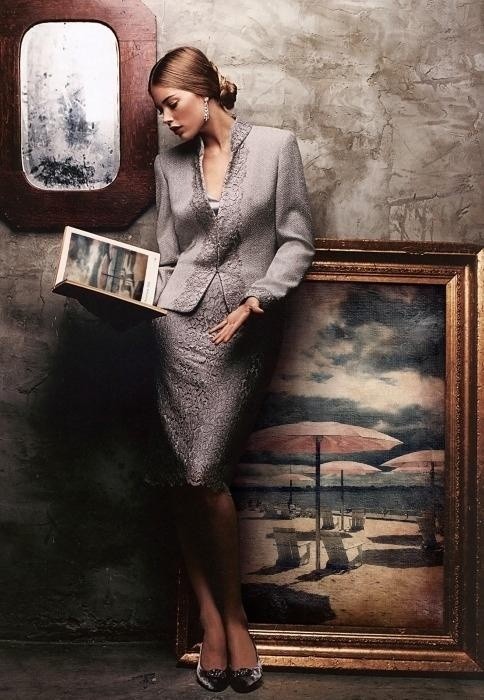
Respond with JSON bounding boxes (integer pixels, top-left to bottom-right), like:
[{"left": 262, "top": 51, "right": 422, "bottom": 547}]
[
  {"left": 197, "top": 644, "right": 230, "bottom": 693},
  {"left": 229, "top": 635, "right": 263, "bottom": 695}
]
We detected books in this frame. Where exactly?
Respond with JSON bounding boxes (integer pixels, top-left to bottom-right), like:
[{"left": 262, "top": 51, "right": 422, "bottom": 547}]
[{"left": 51, "top": 225, "right": 168, "bottom": 332}]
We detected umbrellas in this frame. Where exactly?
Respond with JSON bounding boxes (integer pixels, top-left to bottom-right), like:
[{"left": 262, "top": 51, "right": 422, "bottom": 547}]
[{"left": 233, "top": 420, "right": 445, "bottom": 572}]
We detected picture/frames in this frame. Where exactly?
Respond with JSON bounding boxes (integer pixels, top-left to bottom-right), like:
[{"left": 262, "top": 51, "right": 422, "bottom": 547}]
[
  {"left": 175, "top": 243, "right": 484, "bottom": 680},
  {"left": 0, "top": 2, "right": 159, "bottom": 232}
]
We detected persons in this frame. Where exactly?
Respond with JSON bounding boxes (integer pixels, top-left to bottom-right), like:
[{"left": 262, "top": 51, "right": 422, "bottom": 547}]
[{"left": 147, "top": 46, "right": 313, "bottom": 693}]
